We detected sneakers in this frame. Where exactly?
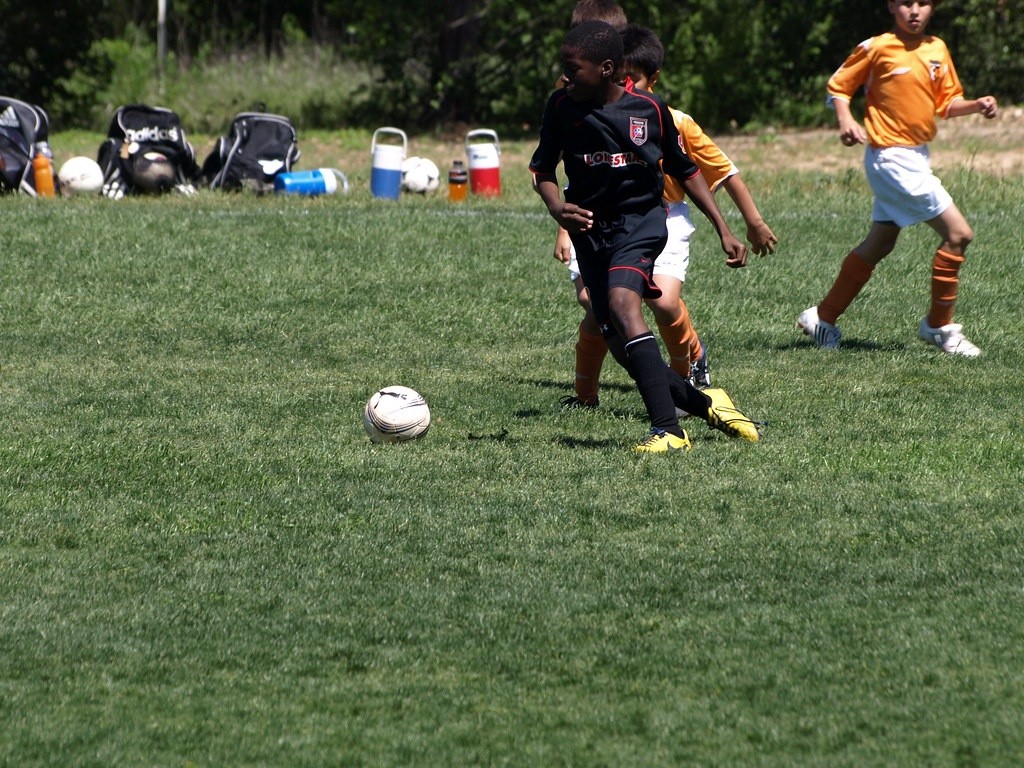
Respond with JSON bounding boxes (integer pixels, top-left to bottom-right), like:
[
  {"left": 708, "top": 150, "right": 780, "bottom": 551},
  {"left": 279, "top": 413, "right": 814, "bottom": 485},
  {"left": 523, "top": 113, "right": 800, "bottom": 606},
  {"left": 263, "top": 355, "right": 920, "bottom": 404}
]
[
  {"left": 702, "top": 388, "right": 768, "bottom": 441},
  {"left": 668, "top": 364, "right": 695, "bottom": 417},
  {"left": 920, "top": 316, "right": 981, "bottom": 357},
  {"left": 797, "top": 306, "right": 841, "bottom": 349},
  {"left": 559, "top": 395, "right": 599, "bottom": 413},
  {"left": 690, "top": 341, "right": 710, "bottom": 387},
  {"left": 634, "top": 427, "right": 691, "bottom": 453}
]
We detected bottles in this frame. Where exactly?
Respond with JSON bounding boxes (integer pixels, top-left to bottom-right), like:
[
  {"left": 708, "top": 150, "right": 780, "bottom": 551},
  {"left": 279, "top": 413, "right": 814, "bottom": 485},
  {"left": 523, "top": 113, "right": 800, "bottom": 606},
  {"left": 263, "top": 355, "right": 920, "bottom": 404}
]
[
  {"left": 448, "top": 161, "right": 469, "bottom": 202},
  {"left": 33, "top": 154, "right": 55, "bottom": 196},
  {"left": 36, "top": 142, "right": 55, "bottom": 180}
]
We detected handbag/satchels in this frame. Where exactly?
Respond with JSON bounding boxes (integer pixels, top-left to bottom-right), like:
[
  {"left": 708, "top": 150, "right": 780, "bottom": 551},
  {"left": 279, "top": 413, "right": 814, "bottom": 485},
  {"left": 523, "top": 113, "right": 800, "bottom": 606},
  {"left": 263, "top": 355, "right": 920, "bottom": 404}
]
[
  {"left": 203, "top": 102, "right": 301, "bottom": 194},
  {"left": 0, "top": 96, "right": 60, "bottom": 198},
  {"left": 97, "top": 104, "right": 203, "bottom": 200}
]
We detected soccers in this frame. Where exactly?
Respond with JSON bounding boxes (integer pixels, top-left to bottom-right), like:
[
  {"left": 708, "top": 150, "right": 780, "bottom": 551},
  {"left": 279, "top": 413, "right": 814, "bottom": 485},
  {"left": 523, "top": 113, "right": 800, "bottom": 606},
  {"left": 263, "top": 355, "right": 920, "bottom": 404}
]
[
  {"left": 59, "top": 155, "right": 104, "bottom": 200},
  {"left": 403, "top": 158, "right": 441, "bottom": 192},
  {"left": 363, "top": 385, "right": 431, "bottom": 445}
]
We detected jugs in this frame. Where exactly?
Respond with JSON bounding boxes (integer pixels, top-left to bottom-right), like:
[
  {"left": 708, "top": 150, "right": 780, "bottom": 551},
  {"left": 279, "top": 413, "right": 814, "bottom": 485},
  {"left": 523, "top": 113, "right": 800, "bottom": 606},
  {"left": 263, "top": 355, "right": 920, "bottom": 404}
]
[
  {"left": 370, "top": 127, "right": 408, "bottom": 200},
  {"left": 275, "top": 167, "right": 349, "bottom": 198},
  {"left": 465, "top": 129, "right": 501, "bottom": 200}
]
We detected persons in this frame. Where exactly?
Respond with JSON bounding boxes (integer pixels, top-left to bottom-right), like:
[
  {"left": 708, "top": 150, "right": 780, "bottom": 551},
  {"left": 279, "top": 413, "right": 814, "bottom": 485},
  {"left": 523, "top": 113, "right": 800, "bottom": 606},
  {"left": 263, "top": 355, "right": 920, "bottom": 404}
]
[
  {"left": 798, "top": 0, "right": 998, "bottom": 359},
  {"left": 529, "top": 0, "right": 779, "bottom": 452}
]
